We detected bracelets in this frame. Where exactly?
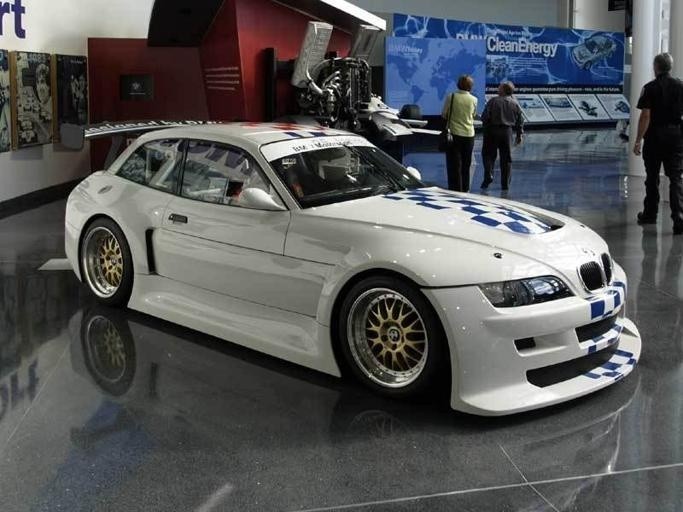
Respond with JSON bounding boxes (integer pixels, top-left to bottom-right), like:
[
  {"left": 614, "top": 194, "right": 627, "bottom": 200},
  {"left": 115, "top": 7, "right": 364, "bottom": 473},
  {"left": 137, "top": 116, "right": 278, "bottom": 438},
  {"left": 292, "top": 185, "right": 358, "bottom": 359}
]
[{"left": 635, "top": 142, "right": 641, "bottom": 146}]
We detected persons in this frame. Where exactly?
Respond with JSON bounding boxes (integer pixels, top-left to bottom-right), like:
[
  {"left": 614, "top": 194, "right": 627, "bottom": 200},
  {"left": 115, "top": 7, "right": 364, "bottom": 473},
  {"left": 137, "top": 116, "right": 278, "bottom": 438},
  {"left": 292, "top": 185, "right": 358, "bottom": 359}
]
[
  {"left": 480, "top": 79, "right": 526, "bottom": 190},
  {"left": 633, "top": 52, "right": 683, "bottom": 235},
  {"left": 33, "top": 64, "right": 53, "bottom": 123},
  {"left": 441, "top": 73, "right": 479, "bottom": 193}
]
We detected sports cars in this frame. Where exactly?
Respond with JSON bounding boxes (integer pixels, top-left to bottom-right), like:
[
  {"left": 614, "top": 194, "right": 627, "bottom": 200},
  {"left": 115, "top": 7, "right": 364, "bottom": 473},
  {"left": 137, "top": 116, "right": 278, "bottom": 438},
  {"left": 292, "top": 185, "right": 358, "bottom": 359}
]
[
  {"left": 63, "top": 119, "right": 642, "bottom": 420},
  {"left": 64, "top": 305, "right": 641, "bottom": 512}
]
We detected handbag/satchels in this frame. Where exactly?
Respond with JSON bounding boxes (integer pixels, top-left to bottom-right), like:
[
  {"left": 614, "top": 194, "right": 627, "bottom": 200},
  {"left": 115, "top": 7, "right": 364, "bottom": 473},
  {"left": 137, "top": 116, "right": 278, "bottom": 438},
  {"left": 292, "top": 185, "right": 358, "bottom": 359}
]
[{"left": 438, "top": 129, "right": 453, "bottom": 151}]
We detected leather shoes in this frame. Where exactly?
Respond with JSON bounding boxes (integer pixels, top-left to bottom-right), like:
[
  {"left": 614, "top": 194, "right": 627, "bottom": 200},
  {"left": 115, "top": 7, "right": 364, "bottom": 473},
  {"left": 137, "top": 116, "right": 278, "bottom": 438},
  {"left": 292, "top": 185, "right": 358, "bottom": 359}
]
[
  {"left": 638, "top": 212, "right": 656, "bottom": 224},
  {"left": 673, "top": 211, "right": 683, "bottom": 235},
  {"left": 480, "top": 176, "right": 494, "bottom": 188}
]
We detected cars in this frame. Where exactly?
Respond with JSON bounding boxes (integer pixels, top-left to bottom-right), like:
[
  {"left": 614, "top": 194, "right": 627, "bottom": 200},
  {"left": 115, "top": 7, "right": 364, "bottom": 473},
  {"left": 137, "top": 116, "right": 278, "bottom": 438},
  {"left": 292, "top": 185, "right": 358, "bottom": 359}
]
[{"left": 570, "top": 37, "right": 616, "bottom": 71}]
